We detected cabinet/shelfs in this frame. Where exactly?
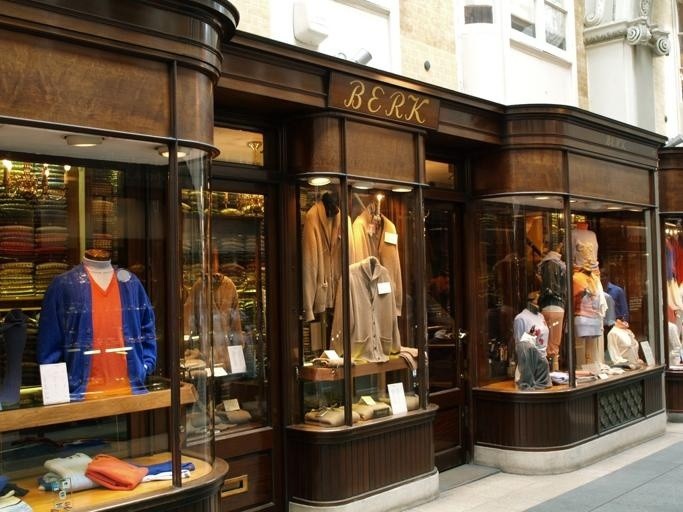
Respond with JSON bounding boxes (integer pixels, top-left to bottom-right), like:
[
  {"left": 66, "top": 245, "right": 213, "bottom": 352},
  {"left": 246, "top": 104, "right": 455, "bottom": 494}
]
[{"left": 181, "top": 209, "right": 265, "bottom": 380}]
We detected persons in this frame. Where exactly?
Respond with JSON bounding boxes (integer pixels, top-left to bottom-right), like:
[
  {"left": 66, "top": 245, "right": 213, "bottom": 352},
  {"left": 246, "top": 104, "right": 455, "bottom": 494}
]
[
  {"left": 37, "top": 250, "right": 160, "bottom": 400},
  {"left": 513, "top": 222, "right": 644, "bottom": 382},
  {"left": 427, "top": 271, "right": 452, "bottom": 297}
]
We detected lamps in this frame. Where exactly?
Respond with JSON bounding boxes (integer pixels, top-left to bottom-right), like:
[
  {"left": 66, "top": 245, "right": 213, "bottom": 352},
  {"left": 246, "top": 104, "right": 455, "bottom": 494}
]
[
  {"left": 0, "top": 215, "right": 70, "bottom": 311},
  {"left": 1, "top": 158, "right": 70, "bottom": 203}
]
